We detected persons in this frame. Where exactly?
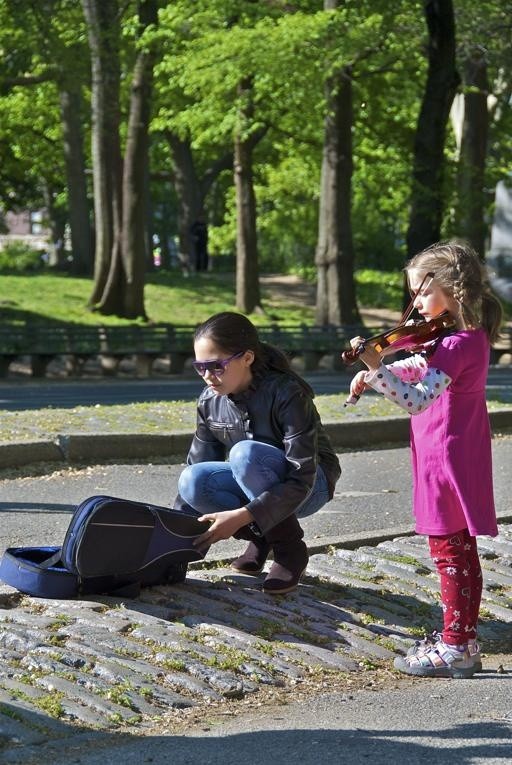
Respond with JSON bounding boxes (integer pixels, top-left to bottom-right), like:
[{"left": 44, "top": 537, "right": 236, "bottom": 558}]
[
  {"left": 350, "top": 241, "right": 502, "bottom": 679},
  {"left": 174, "top": 313, "right": 343, "bottom": 594}
]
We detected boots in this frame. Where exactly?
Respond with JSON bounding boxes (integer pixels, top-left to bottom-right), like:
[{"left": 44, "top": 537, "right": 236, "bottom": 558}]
[
  {"left": 230, "top": 521, "right": 271, "bottom": 573},
  {"left": 262, "top": 515, "right": 309, "bottom": 593}
]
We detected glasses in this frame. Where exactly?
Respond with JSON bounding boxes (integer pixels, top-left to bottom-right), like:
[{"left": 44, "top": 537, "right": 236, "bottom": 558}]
[{"left": 192, "top": 351, "right": 241, "bottom": 378}]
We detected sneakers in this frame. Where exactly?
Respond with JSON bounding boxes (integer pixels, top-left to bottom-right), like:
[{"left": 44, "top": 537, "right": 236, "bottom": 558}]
[
  {"left": 394, "top": 640, "right": 476, "bottom": 680},
  {"left": 409, "top": 635, "right": 482, "bottom": 673}
]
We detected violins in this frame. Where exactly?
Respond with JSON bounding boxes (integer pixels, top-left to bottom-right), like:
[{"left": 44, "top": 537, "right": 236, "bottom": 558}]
[{"left": 342, "top": 310, "right": 460, "bottom": 366}]
